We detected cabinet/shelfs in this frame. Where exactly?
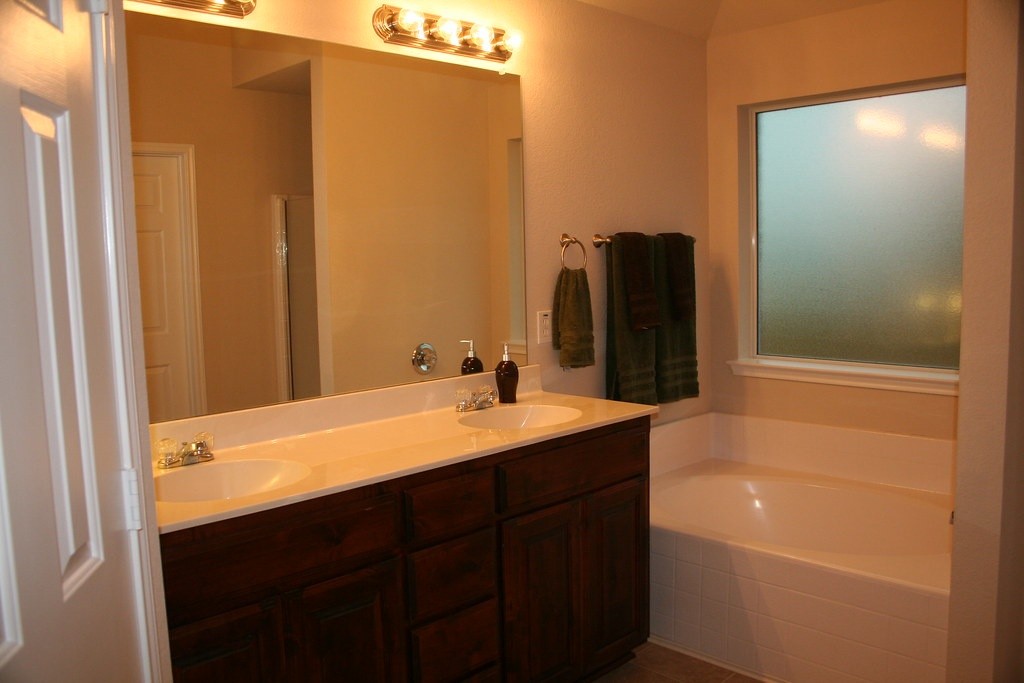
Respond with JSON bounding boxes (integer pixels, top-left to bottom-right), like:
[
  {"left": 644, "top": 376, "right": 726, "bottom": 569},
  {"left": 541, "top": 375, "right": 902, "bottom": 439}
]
[{"left": 160, "top": 414, "right": 652, "bottom": 683}]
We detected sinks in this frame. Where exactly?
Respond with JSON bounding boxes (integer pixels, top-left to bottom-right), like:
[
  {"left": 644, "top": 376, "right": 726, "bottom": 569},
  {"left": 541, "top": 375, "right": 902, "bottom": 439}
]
[
  {"left": 457, "top": 401, "right": 584, "bottom": 430},
  {"left": 152, "top": 456, "right": 307, "bottom": 503}
]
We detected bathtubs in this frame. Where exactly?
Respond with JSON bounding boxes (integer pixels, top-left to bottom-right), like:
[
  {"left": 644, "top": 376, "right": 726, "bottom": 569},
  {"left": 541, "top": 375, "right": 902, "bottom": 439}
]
[{"left": 648, "top": 456, "right": 955, "bottom": 682}]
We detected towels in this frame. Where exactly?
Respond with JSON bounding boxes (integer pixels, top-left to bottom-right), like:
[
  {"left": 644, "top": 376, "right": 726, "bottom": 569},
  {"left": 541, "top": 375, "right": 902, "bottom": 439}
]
[
  {"left": 550, "top": 264, "right": 596, "bottom": 371},
  {"left": 605, "top": 230, "right": 700, "bottom": 406}
]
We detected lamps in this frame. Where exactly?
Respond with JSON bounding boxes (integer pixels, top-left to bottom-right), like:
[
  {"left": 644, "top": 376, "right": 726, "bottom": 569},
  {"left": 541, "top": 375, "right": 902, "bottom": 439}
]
[
  {"left": 130, "top": 0, "right": 256, "bottom": 19},
  {"left": 372, "top": 2, "right": 527, "bottom": 64}
]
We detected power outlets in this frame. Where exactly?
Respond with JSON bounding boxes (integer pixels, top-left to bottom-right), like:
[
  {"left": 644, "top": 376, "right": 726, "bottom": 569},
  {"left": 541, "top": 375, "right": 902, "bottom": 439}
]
[{"left": 536, "top": 309, "right": 554, "bottom": 346}]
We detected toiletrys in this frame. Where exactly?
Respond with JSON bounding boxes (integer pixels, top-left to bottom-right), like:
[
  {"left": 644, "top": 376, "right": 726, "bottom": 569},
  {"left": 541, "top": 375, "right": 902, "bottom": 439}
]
[
  {"left": 454, "top": 337, "right": 484, "bottom": 375},
  {"left": 494, "top": 340, "right": 520, "bottom": 404}
]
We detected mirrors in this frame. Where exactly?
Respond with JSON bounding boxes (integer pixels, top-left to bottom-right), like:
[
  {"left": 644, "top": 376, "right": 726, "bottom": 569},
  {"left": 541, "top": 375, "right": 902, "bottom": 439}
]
[{"left": 123, "top": 7, "right": 530, "bottom": 429}]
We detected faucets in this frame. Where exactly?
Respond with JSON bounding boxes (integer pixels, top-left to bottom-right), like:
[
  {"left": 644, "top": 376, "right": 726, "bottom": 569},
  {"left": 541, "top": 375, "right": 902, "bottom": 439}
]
[
  {"left": 171, "top": 440, "right": 204, "bottom": 463},
  {"left": 473, "top": 389, "right": 499, "bottom": 409}
]
